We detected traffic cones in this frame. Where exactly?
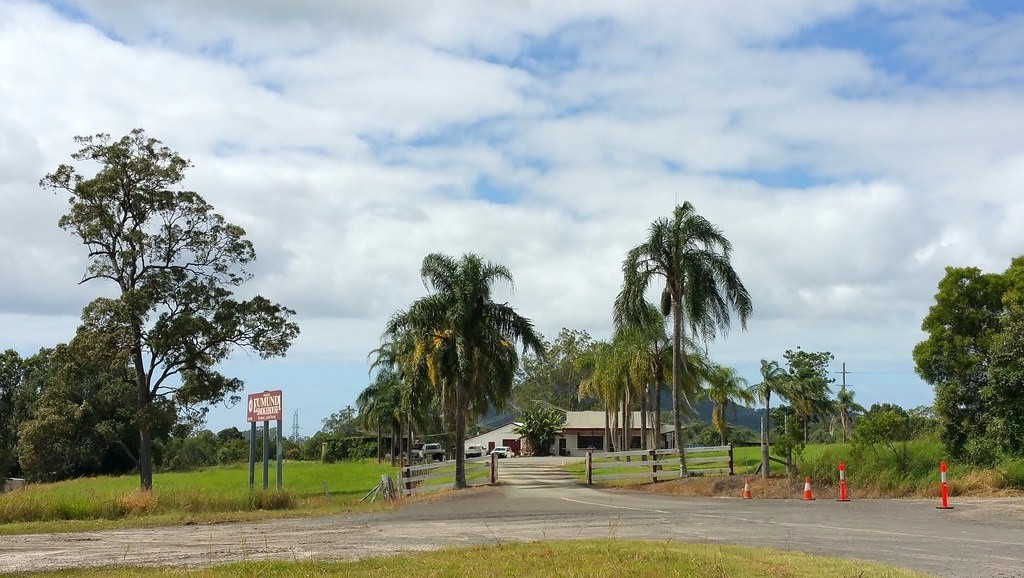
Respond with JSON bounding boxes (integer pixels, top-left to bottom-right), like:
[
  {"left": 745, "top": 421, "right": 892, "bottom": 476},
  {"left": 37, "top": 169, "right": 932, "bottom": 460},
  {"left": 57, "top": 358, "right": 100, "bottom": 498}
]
[
  {"left": 742, "top": 477, "right": 753, "bottom": 499},
  {"left": 800, "top": 476, "right": 817, "bottom": 500}
]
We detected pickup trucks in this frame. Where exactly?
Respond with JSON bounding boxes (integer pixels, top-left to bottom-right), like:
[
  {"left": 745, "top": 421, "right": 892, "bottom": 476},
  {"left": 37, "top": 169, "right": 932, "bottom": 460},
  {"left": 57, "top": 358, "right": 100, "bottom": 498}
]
[
  {"left": 490, "top": 447, "right": 515, "bottom": 458},
  {"left": 465, "top": 445, "right": 487, "bottom": 458}
]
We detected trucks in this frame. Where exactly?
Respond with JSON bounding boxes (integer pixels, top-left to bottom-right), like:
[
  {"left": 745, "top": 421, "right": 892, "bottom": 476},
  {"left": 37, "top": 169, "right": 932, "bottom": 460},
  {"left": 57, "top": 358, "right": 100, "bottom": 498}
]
[{"left": 418, "top": 443, "right": 446, "bottom": 461}]
